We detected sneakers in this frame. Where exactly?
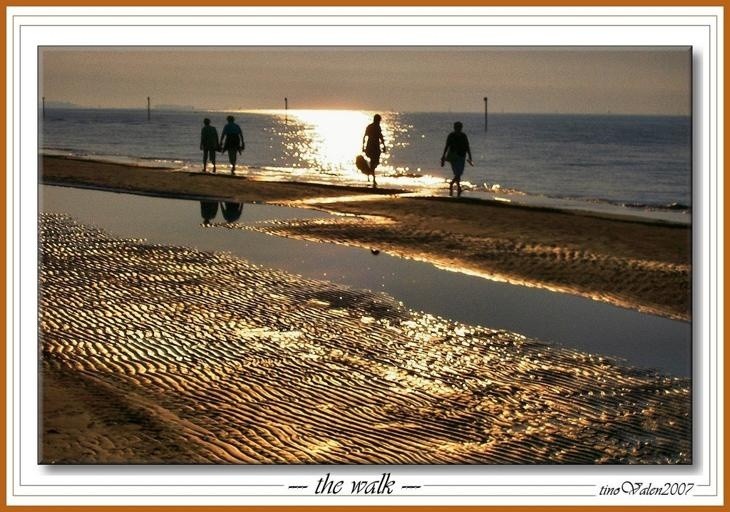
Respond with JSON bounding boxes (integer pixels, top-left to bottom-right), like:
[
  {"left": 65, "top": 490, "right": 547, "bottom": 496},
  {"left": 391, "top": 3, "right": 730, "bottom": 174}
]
[
  {"left": 441, "top": 157, "right": 445, "bottom": 167},
  {"left": 467, "top": 159, "right": 474, "bottom": 166}
]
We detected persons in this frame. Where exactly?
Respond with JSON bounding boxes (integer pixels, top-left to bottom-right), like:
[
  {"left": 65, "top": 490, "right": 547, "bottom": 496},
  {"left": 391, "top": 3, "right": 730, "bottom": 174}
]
[
  {"left": 220, "top": 201, "right": 243, "bottom": 223},
  {"left": 200, "top": 200, "right": 218, "bottom": 224},
  {"left": 362, "top": 113, "right": 385, "bottom": 185},
  {"left": 441, "top": 121, "right": 472, "bottom": 194},
  {"left": 200, "top": 118, "right": 218, "bottom": 172},
  {"left": 220, "top": 115, "right": 245, "bottom": 172}
]
